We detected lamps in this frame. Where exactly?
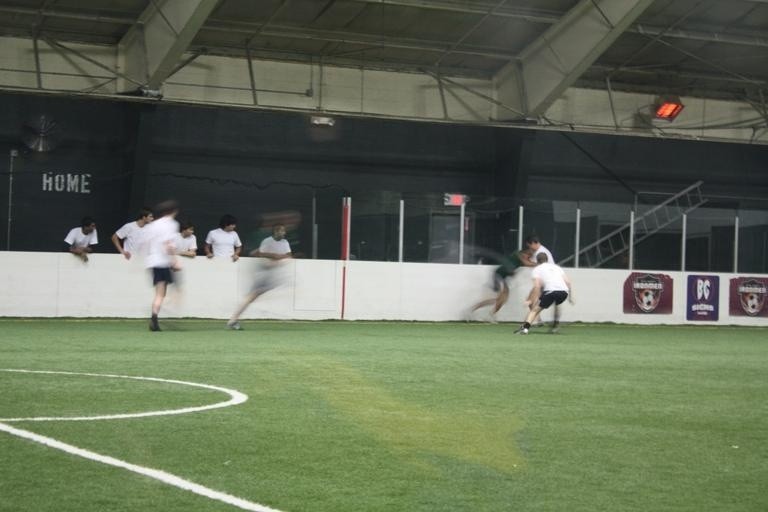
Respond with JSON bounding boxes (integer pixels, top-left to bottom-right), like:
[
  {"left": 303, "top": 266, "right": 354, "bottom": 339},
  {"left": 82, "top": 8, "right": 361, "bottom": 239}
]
[
  {"left": 307, "top": 115, "right": 335, "bottom": 128},
  {"left": 138, "top": 86, "right": 161, "bottom": 100},
  {"left": 651, "top": 92, "right": 684, "bottom": 122}
]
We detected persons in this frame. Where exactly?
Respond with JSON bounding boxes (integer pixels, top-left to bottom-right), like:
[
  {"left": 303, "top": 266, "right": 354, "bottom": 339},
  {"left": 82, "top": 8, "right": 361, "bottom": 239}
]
[
  {"left": 227, "top": 225, "right": 294, "bottom": 330},
  {"left": 139, "top": 198, "right": 183, "bottom": 332},
  {"left": 203, "top": 211, "right": 243, "bottom": 262},
  {"left": 62, "top": 215, "right": 99, "bottom": 262},
  {"left": 521, "top": 236, "right": 555, "bottom": 328},
  {"left": 177, "top": 219, "right": 200, "bottom": 257},
  {"left": 462, "top": 246, "right": 538, "bottom": 324},
  {"left": 110, "top": 206, "right": 155, "bottom": 259},
  {"left": 515, "top": 252, "right": 577, "bottom": 335}
]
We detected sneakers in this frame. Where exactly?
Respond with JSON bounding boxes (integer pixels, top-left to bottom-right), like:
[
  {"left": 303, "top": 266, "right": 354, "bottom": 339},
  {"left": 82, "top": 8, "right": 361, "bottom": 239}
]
[
  {"left": 149, "top": 314, "right": 160, "bottom": 331},
  {"left": 535, "top": 322, "right": 545, "bottom": 327},
  {"left": 461, "top": 309, "right": 470, "bottom": 322},
  {"left": 550, "top": 323, "right": 561, "bottom": 333},
  {"left": 484, "top": 316, "right": 497, "bottom": 324},
  {"left": 228, "top": 319, "right": 242, "bottom": 329},
  {"left": 514, "top": 326, "right": 529, "bottom": 335}
]
